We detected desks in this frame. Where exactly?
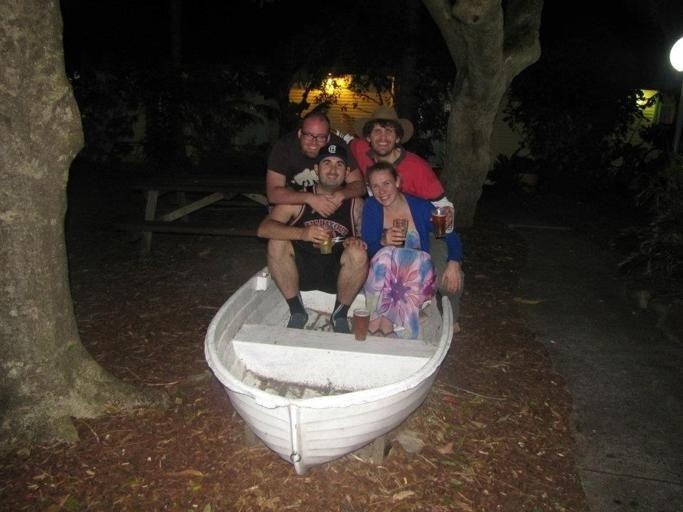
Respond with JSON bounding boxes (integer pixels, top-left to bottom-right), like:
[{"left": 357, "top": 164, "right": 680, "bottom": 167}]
[{"left": 129, "top": 177, "right": 274, "bottom": 270}]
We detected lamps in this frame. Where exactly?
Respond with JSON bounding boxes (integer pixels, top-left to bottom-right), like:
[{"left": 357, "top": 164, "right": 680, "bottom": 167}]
[{"left": 669, "top": 38, "right": 683, "bottom": 71}]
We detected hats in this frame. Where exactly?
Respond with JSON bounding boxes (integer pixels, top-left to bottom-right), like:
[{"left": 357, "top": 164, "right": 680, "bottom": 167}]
[
  {"left": 354, "top": 107, "right": 414, "bottom": 144},
  {"left": 316, "top": 143, "right": 347, "bottom": 162}
]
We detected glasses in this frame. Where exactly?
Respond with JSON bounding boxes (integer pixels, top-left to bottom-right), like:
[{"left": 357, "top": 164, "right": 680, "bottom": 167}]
[{"left": 301, "top": 130, "right": 329, "bottom": 143}]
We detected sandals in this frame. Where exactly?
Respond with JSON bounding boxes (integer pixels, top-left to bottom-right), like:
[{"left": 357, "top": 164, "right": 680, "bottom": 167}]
[{"left": 368, "top": 328, "right": 397, "bottom": 337}]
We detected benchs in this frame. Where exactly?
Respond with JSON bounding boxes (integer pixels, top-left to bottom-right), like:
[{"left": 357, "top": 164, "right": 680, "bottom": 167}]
[{"left": 99, "top": 214, "right": 258, "bottom": 269}]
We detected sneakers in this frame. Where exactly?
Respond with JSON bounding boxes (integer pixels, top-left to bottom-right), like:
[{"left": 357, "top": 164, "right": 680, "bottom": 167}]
[
  {"left": 287, "top": 311, "right": 308, "bottom": 329},
  {"left": 330, "top": 315, "right": 350, "bottom": 332}
]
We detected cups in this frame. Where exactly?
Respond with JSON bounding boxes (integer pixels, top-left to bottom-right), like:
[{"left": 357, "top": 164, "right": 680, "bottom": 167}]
[
  {"left": 431, "top": 207, "right": 447, "bottom": 238},
  {"left": 320, "top": 226, "right": 333, "bottom": 254},
  {"left": 354, "top": 308, "right": 370, "bottom": 341},
  {"left": 392, "top": 219, "right": 407, "bottom": 247}
]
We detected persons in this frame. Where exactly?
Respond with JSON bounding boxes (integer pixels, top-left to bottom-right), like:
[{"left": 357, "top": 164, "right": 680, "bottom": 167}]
[
  {"left": 265, "top": 112, "right": 366, "bottom": 218},
  {"left": 348, "top": 118, "right": 455, "bottom": 235},
  {"left": 256, "top": 143, "right": 369, "bottom": 335},
  {"left": 360, "top": 160, "right": 462, "bottom": 340}
]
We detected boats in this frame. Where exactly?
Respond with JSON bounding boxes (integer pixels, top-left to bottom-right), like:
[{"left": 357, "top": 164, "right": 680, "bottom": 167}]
[{"left": 203, "top": 244, "right": 454, "bottom": 476}]
